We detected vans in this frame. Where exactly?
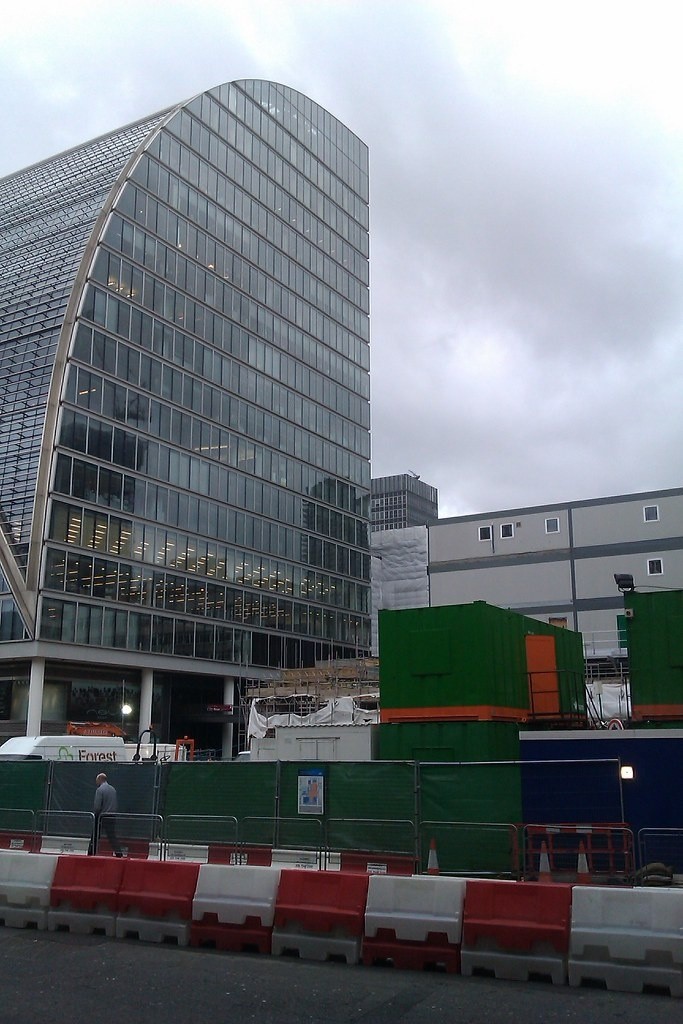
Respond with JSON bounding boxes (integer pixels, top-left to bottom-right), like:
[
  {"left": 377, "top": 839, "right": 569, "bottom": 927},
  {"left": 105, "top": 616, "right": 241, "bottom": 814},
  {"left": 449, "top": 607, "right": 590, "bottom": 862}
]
[{"left": 0, "top": 736, "right": 126, "bottom": 761}]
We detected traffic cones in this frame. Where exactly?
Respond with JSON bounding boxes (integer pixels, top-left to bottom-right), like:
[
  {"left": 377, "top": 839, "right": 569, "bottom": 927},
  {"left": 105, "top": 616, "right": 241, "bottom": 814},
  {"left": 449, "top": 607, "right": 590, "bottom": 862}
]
[
  {"left": 537, "top": 841, "right": 553, "bottom": 882},
  {"left": 577, "top": 840, "right": 591, "bottom": 884},
  {"left": 427, "top": 838, "right": 441, "bottom": 875}
]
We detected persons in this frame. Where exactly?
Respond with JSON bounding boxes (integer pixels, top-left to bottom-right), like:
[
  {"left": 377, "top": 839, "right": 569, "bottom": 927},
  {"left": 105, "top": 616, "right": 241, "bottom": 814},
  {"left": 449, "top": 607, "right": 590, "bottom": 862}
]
[{"left": 87, "top": 773, "right": 123, "bottom": 858}]
